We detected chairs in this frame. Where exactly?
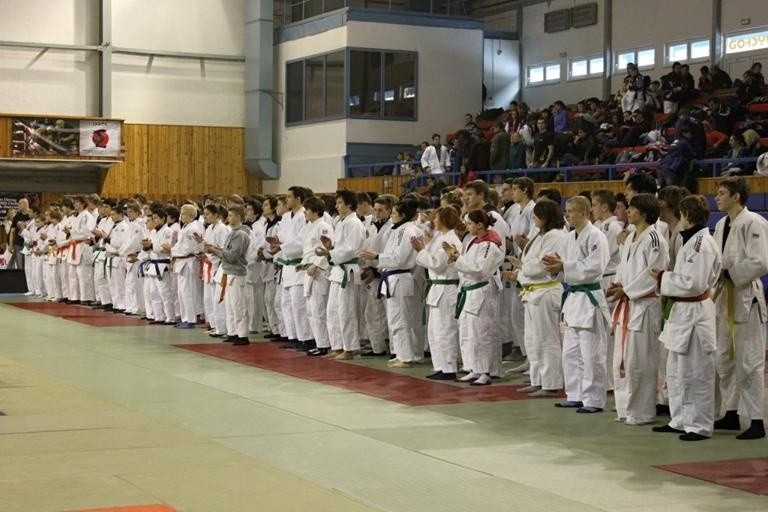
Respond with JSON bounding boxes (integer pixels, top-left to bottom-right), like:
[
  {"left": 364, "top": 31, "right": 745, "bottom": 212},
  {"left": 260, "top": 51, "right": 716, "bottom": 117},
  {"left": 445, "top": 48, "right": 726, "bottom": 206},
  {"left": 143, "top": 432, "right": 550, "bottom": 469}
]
[{"left": 447, "top": 95, "right": 768, "bottom": 174}]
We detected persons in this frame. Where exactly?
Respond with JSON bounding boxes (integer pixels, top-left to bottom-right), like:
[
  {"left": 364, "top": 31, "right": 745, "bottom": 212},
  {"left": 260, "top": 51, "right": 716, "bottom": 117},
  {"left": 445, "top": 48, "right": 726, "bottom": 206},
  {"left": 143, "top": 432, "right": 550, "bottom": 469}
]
[
  {"left": 382, "top": 59, "right": 768, "bottom": 195},
  {"left": 1, "top": 188, "right": 768, "bottom": 442}
]
